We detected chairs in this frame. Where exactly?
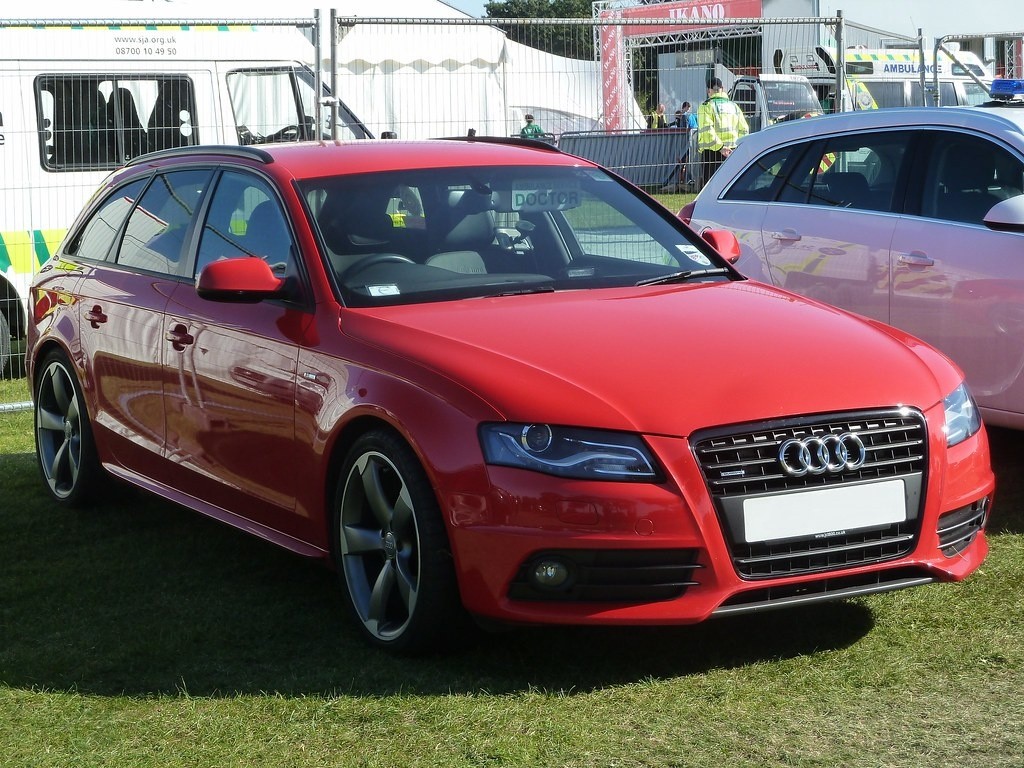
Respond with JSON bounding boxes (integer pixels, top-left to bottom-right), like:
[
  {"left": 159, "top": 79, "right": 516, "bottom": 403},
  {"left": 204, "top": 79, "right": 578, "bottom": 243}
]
[
  {"left": 769, "top": 162, "right": 1023, "bottom": 230},
  {"left": 128, "top": 188, "right": 537, "bottom": 279},
  {"left": 99, "top": 88, "right": 154, "bottom": 163}
]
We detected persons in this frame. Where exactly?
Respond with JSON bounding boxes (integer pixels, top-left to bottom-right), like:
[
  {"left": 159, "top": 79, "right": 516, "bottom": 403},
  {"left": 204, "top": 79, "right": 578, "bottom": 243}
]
[
  {"left": 697, "top": 77, "right": 750, "bottom": 191},
  {"left": 647, "top": 102, "right": 697, "bottom": 129},
  {"left": 521, "top": 114, "right": 544, "bottom": 139}
]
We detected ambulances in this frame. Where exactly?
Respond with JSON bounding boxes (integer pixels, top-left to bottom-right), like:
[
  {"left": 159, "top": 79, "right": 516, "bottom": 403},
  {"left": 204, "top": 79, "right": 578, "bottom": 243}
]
[
  {"left": 816, "top": 44, "right": 999, "bottom": 165},
  {"left": 0, "top": 23, "right": 530, "bottom": 379}
]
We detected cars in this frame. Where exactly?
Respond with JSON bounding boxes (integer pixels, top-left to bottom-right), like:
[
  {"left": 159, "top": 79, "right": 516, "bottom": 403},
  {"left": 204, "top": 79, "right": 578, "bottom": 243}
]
[
  {"left": 727, "top": 73, "right": 826, "bottom": 134},
  {"left": 21, "top": 136, "right": 996, "bottom": 661},
  {"left": 676, "top": 78, "right": 1024, "bottom": 437}
]
